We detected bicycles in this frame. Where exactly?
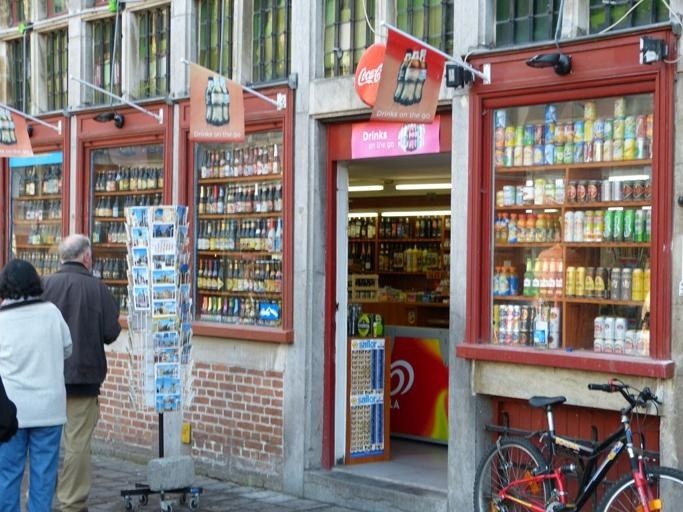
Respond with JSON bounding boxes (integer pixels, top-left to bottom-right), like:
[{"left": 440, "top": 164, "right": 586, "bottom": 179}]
[{"left": 469, "top": 377, "right": 682, "bottom": 512}]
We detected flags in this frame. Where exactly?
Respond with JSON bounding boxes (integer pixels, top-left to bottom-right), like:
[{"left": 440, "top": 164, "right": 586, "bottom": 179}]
[
  {"left": 187, "top": 62, "right": 246, "bottom": 144},
  {"left": 0, "top": 104, "right": 35, "bottom": 160},
  {"left": 369, "top": 29, "right": 447, "bottom": 125}
]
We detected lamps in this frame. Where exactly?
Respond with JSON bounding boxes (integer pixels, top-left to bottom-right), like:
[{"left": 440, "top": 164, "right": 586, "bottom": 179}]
[
  {"left": 640, "top": 36, "right": 669, "bottom": 66},
  {"left": 525, "top": 52, "right": 572, "bottom": 76},
  {"left": 445, "top": 63, "right": 476, "bottom": 88},
  {"left": 93, "top": 111, "right": 124, "bottom": 128}
]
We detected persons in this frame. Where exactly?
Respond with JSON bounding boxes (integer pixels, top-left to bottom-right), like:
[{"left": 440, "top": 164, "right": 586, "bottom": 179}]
[
  {"left": 31, "top": 231, "right": 122, "bottom": 511},
  {"left": 0, "top": 257, "right": 73, "bottom": 511}
]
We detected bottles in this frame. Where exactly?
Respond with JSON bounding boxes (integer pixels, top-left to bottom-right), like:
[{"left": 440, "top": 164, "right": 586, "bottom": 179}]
[
  {"left": 11, "top": 166, "right": 63, "bottom": 277},
  {"left": 1, "top": 109, "right": 19, "bottom": 146},
  {"left": 394, "top": 46, "right": 429, "bottom": 106},
  {"left": 494, "top": 213, "right": 562, "bottom": 299},
  {"left": 93, "top": 167, "right": 163, "bottom": 311},
  {"left": 349, "top": 217, "right": 444, "bottom": 272},
  {"left": 196, "top": 142, "right": 282, "bottom": 324},
  {"left": 204, "top": 74, "right": 230, "bottom": 125}
]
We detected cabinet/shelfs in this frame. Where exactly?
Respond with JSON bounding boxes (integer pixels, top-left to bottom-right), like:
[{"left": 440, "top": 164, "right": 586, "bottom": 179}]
[
  {"left": 494, "top": 158, "right": 652, "bottom": 355},
  {"left": 10, "top": 195, "right": 62, "bottom": 279},
  {"left": 188, "top": 174, "right": 283, "bottom": 326},
  {"left": 93, "top": 189, "right": 167, "bottom": 315},
  {"left": 349, "top": 209, "right": 450, "bottom": 327}
]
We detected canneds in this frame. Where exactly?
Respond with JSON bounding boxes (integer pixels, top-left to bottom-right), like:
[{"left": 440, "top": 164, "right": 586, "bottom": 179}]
[
  {"left": 565, "top": 209, "right": 653, "bottom": 242},
  {"left": 592, "top": 315, "right": 650, "bottom": 356},
  {"left": 496, "top": 176, "right": 564, "bottom": 208},
  {"left": 563, "top": 266, "right": 650, "bottom": 302},
  {"left": 492, "top": 304, "right": 560, "bottom": 350},
  {"left": 494, "top": 96, "right": 654, "bottom": 168},
  {"left": 568, "top": 178, "right": 652, "bottom": 203}
]
